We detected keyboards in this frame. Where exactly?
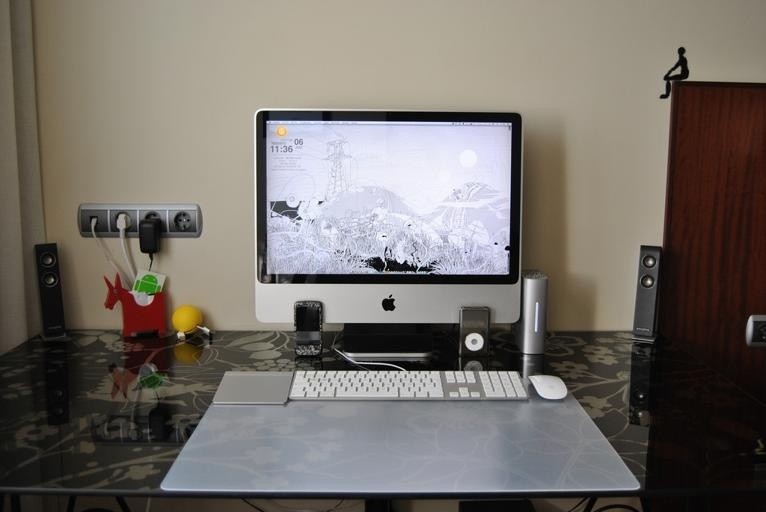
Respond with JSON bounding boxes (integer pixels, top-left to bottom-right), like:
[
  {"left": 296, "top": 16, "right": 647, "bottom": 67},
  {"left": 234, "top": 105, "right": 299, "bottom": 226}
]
[{"left": 290, "top": 370, "right": 529, "bottom": 403}]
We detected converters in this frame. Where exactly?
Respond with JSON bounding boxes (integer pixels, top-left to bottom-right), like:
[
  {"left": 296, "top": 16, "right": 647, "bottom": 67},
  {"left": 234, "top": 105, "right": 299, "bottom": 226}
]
[{"left": 139, "top": 219, "right": 160, "bottom": 254}]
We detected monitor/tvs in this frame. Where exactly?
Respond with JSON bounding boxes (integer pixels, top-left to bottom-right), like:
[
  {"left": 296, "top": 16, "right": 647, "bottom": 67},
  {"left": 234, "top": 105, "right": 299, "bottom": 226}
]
[{"left": 253, "top": 107, "right": 523, "bottom": 361}]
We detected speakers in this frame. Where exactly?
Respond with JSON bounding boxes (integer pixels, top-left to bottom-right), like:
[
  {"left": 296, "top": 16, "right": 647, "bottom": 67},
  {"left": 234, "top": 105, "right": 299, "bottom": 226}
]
[
  {"left": 633, "top": 242, "right": 657, "bottom": 344},
  {"left": 522, "top": 267, "right": 549, "bottom": 357},
  {"left": 461, "top": 305, "right": 492, "bottom": 361},
  {"left": 35, "top": 242, "right": 67, "bottom": 341}
]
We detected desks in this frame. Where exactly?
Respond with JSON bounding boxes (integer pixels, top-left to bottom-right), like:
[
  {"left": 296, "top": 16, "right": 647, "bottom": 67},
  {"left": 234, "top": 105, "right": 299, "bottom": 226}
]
[{"left": 0, "top": 327, "right": 766, "bottom": 501}]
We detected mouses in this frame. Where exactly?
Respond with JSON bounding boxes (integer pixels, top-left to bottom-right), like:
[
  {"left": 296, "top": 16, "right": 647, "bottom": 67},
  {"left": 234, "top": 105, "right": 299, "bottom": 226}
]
[{"left": 531, "top": 375, "right": 570, "bottom": 400}]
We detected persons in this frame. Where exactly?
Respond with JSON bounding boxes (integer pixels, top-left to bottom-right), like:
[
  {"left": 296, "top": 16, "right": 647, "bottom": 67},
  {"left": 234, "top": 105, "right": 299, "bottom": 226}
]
[{"left": 659, "top": 47, "right": 690, "bottom": 100}]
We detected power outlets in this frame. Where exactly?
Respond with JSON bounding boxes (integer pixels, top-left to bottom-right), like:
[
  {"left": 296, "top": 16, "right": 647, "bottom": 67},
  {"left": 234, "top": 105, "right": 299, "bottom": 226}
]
[{"left": 76, "top": 202, "right": 203, "bottom": 239}]
